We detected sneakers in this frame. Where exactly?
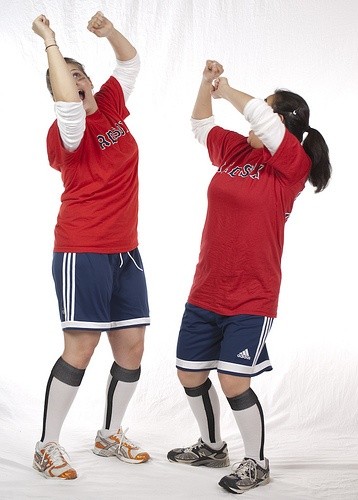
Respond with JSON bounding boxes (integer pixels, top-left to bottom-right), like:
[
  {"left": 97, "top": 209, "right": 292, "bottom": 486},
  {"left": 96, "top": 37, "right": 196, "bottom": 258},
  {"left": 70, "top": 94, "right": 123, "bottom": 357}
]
[
  {"left": 220, "top": 458, "right": 271, "bottom": 494},
  {"left": 92, "top": 429, "right": 151, "bottom": 464},
  {"left": 167, "top": 438, "right": 230, "bottom": 468},
  {"left": 31, "top": 441, "right": 78, "bottom": 482}
]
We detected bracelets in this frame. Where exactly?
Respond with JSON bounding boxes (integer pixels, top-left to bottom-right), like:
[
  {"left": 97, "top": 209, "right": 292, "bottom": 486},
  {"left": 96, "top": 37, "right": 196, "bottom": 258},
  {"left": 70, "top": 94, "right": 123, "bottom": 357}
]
[{"left": 44, "top": 44, "right": 59, "bottom": 52}]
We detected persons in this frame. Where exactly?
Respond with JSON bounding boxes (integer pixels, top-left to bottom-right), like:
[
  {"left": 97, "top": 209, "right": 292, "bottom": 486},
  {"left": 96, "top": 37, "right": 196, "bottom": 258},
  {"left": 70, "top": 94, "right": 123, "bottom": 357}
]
[
  {"left": 32, "top": 11, "right": 151, "bottom": 480},
  {"left": 166, "top": 58, "right": 333, "bottom": 494}
]
